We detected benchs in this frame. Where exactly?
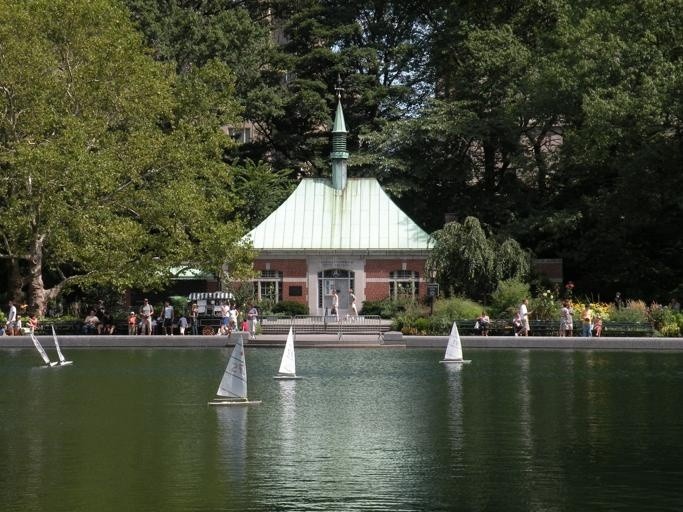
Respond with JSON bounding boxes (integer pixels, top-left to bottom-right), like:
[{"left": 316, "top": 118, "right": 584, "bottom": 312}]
[
  {"left": 0, "top": 320, "right": 129, "bottom": 333},
  {"left": 457, "top": 318, "right": 655, "bottom": 337}
]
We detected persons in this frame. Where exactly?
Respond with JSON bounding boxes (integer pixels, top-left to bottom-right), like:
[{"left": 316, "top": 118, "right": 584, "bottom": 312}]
[
  {"left": 28, "top": 313, "right": 38, "bottom": 335},
  {"left": 669, "top": 298, "right": 679, "bottom": 312},
  {"left": 476, "top": 310, "right": 492, "bottom": 337},
  {"left": 330, "top": 289, "right": 339, "bottom": 323},
  {"left": 6, "top": 300, "right": 17, "bottom": 336},
  {"left": 555, "top": 297, "right": 603, "bottom": 337},
  {"left": 513, "top": 299, "right": 531, "bottom": 336},
  {"left": 0, "top": 322, "right": 7, "bottom": 336},
  {"left": 212, "top": 297, "right": 259, "bottom": 340},
  {"left": 159, "top": 298, "right": 175, "bottom": 336},
  {"left": 511, "top": 310, "right": 526, "bottom": 337},
  {"left": 138, "top": 297, "right": 154, "bottom": 336},
  {"left": 80, "top": 309, "right": 99, "bottom": 335},
  {"left": 174, "top": 312, "right": 189, "bottom": 336},
  {"left": 187, "top": 303, "right": 200, "bottom": 336},
  {"left": 126, "top": 311, "right": 138, "bottom": 334},
  {"left": 615, "top": 291, "right": 624, "bottom": 312},
  {"left": 348, "top": 295, "right": 358, "bottom": 320},
  {"left": 347, "top": 287, "right": 355, "bottom": 308},
  {"left": 13, "top": 315, "right": 22, "bottom": 337},
  {"left": 96, "top": 311, "right": 116, "bottom": 335},
  {"left": 150, "top": 309, "right": 157, "bottom": 335}
]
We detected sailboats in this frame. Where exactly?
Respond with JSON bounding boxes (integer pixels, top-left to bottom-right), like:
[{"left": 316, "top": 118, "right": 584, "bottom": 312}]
[
  {"left": 208, "top": 334, "right": 261, "bottom": 405},
  {"left": 440, "top": 321, "right": 471, "bottom": 363},
  {"left": 29, "top": 324, "right": 73, "bottom": 369},
  {"left": 273, "top": 325, "right": 301, "bottom": 379}
]
